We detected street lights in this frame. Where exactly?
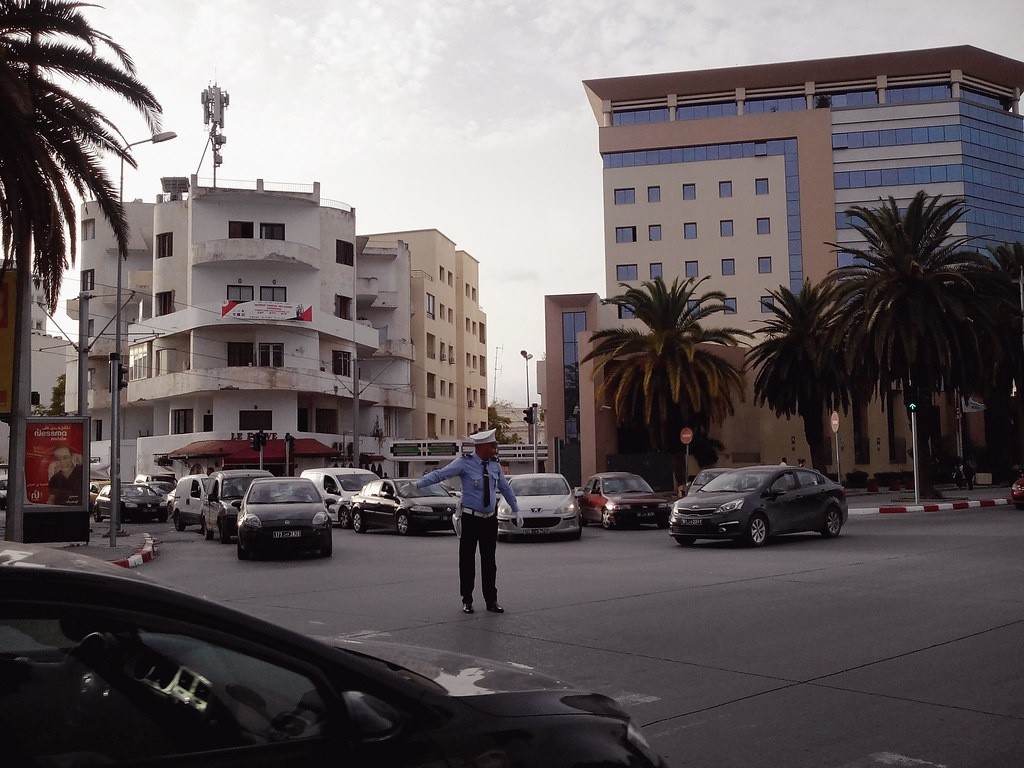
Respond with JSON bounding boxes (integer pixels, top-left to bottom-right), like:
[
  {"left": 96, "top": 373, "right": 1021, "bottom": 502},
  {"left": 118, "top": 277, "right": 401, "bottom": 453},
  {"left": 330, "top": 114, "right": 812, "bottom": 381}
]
[
  {"left": 108, "top": 130, "right": 178, "bottom": 538},
  {"left": 106, "top": 352, "right": 121, "bottom": 550},
  {"left": 519, "top": 349, "right": 532, "bottom": 408},
  {"left": 340, "top": 429, "right": 353, "bottom": 467}
]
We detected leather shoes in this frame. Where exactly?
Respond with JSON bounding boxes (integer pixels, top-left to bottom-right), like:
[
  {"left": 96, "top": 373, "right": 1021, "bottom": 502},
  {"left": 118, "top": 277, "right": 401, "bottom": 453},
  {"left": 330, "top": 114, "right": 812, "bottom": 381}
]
[
  {"left": 486, "top": 602, "right": 504, "bottom": 612},
  {"left": 463, "top": 602, "right": 474, "bottom": 614}
]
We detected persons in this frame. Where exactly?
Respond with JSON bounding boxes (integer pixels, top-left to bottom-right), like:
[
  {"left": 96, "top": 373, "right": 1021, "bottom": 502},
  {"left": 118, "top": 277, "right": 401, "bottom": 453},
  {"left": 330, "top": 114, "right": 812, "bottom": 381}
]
[
  {"left": 47, "top": 444, "right": 82, "bottom": 506},
  {"left": 798, "top": 458, "right": 806, "bottom": 467},
  {"left": 962, "top": 463, "right": 976, "bottom": 490},
  {"left": 400, "top": 428, "right": 524, "bottom": 614},
  {"left": 779, "top": 457, "right": 791, "bottom": 481},
  {"left": 382, "top": 472, "right": 389, "bottom": 479},
  {"left": 951, "top": 464, "right": 962, "bottom": 488}
]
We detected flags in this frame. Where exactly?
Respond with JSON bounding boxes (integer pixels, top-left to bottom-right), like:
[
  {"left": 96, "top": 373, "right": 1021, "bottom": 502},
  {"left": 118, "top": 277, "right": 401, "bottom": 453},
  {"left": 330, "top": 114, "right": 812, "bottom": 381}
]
[{"left": 961, "top": 394, "right": 986, "bottom": 413}]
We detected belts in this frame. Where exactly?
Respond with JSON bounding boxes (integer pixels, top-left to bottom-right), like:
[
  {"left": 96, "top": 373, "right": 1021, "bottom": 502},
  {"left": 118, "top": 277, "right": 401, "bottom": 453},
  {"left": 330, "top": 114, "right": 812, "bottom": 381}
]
[{"left": 464, "top": 507, "right": 493, "bottom": 518}]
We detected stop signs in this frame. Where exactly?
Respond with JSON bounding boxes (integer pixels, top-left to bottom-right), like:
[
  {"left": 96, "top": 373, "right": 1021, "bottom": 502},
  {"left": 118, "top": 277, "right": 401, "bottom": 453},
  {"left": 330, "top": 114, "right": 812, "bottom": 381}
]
[
  {"left": 830, "top": 411, "right": 840, "bottom": 433},
  {"left": 679, "top": 427, "right": 692, "bottom": 445}
]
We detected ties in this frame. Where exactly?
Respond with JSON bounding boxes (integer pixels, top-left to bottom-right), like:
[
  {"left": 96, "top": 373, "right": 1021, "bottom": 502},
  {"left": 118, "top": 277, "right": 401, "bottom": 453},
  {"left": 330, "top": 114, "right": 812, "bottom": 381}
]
[{"left": 481, "top": 461, "right": 490, "bottom": 508}]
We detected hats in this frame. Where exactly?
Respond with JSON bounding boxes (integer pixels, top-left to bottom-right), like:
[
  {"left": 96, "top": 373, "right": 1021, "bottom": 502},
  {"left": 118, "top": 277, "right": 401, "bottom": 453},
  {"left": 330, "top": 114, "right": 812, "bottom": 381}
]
[{"left": 470, "top": 428, "right": 496, "bottom": 444}]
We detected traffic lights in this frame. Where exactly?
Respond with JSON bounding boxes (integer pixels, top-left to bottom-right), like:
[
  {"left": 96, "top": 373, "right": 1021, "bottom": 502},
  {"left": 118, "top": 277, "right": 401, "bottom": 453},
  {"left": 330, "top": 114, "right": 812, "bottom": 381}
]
[
  {"left": 108, "top": 362, "right": 128, "bottom": 393},
  {"left": 902, "top": 385, "right": 919, "bottom": 411},
  {"left": 337, "top": 442, "right": 344, "bottom": 453},
  {"left": 249, "top": 434, "right": 260, "bottom": 452},
  {"left": 346, "top": 441, "right": 354, "bottom": 454},
  {"left": 289, "top": 436, "right": 296, "bottom": 452}
]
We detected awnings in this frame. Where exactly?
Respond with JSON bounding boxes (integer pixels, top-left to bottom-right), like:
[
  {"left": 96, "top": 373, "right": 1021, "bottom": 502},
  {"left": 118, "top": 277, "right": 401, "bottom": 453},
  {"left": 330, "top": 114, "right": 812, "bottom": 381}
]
[
  {"left": 221, "top": 438, "right": 341, "bottom": 463},
  {"left": 168, "top": 440, "right": 251, "bottom": 459}
]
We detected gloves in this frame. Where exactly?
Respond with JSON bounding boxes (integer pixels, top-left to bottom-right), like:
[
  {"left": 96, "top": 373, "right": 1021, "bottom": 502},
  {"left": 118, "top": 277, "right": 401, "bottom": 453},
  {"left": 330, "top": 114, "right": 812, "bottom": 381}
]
[
  {"left": 516, "top": 510, "right": 525, "bottom": 527},
  {"left": 401, "top": 480, "right": 417, "bottom": 496}
]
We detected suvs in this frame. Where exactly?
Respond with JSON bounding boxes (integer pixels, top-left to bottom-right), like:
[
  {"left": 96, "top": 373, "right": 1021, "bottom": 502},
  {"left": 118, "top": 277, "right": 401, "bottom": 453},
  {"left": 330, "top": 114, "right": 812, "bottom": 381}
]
[{"left": 297, "top": 467, "right": 380, "bottom": 529}]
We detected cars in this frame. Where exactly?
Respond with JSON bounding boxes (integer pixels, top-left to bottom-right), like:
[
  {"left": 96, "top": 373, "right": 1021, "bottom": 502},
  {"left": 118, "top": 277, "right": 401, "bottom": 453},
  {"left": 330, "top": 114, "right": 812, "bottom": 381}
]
[
  {"left": 91, "top": 484, "right": 170, "bottom": 523},
  {"left": 669, "top": 464, "right": 849, "bottom": 547},
  {"left": 133, "top": 474, "right": 180, "bottom": 517},
  {"left": 685, "top": 467, "right": 747, "bottom": 497},
  {"left": 88, "top": 481, "right": 113, "bottom": 512},
  {"left": 576, "top": 471, "right": 671, "bottom": 530},
  {"left": 495, "top": 472, "right": 583, "bottom": 539},
  {"left": 1, "top": 539, "right": 666, "bottom": 767},
  {"left": 200, "top": 469, "right": 279, "bottom": 544},
  {"left": 171, "top": 474, "right": 212, "bottom": 534},
  {"left": 349, "top": 478, "right": 461, "bottom": 536},
  {"left": 227, "top": 476, "right": 337, "bottom": 559},
  {"left": 0, "top": 476, "right": 8, "bottom": 511},
  {"left": 492, "top": 475, "right": 515, "bottom": 503}
]
